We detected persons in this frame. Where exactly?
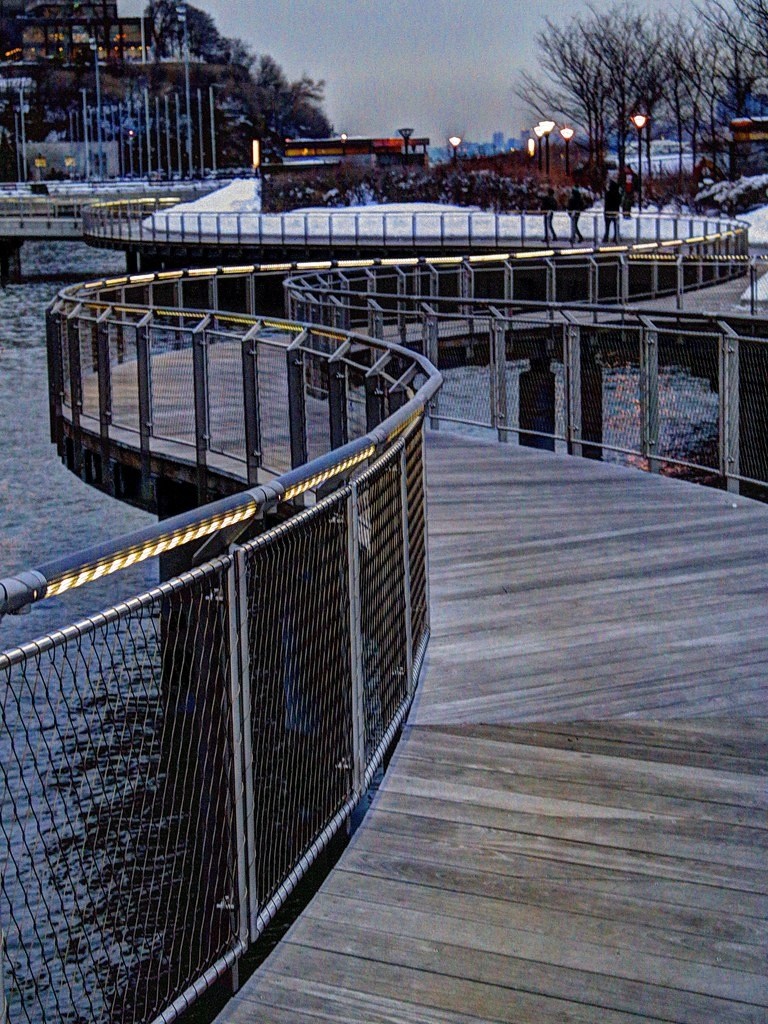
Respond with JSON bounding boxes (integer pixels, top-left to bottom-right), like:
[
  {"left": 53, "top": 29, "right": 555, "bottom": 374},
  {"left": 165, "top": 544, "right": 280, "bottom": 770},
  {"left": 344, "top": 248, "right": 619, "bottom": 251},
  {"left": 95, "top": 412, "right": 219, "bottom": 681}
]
[
  {"left": 539, "top": 188, "right": 559, "bottom": 241},
  {"left": 601, "top": 169, "right": 623, "bottom": 243},
  {"left": 566, "top": 189, "right": 586, "bottom": 243},
  {"left": 620, "top": 164, "right": 638, "bottom": 220}
]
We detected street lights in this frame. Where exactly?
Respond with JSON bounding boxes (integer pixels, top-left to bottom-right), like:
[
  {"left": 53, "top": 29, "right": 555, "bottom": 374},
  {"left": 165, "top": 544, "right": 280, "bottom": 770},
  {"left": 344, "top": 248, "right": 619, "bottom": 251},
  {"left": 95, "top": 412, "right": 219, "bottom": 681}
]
[
  {"left": 559, "top": 127, "right": 574, "bottom": 177},
  {"left": 628, "top": 115, "right": 651, "bottom": 212},
  {"left": 534, "top": 121, "right": 556, "bottom": 176},
  {"left": 450, "top": 137, "right": 462, "bottom": 164}
]
